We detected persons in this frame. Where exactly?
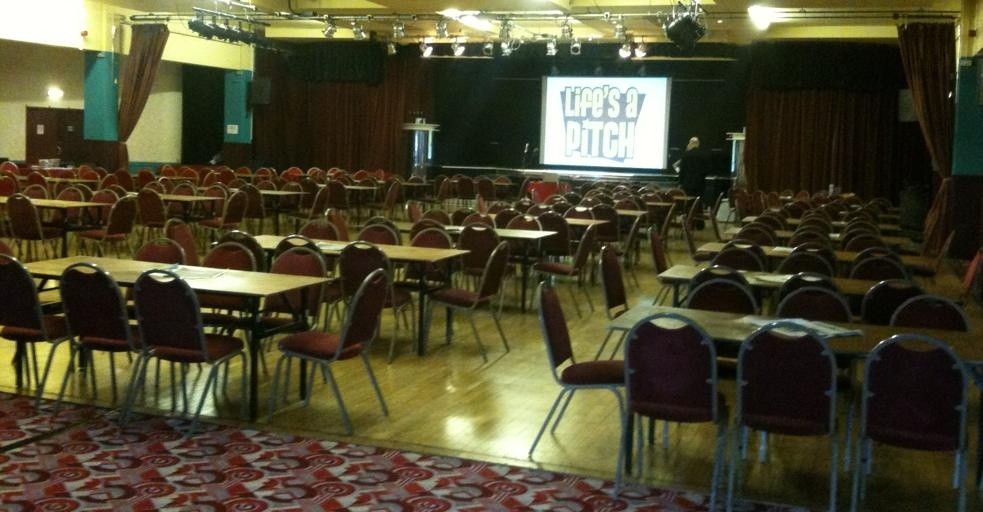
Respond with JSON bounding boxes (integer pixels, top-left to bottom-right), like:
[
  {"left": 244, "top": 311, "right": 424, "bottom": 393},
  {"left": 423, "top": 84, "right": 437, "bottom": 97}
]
[{"left": 678, "top": 137, "right": 708, "bottom": 226}]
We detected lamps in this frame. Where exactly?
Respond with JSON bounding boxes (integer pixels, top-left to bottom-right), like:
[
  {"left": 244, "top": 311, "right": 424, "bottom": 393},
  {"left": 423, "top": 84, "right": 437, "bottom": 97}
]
[
  {"left": 662, "top": 0, "right": 709, "bottom": 50},
  {"left": 188, "top": 7, "right": 281, "bottom": 55}
]
